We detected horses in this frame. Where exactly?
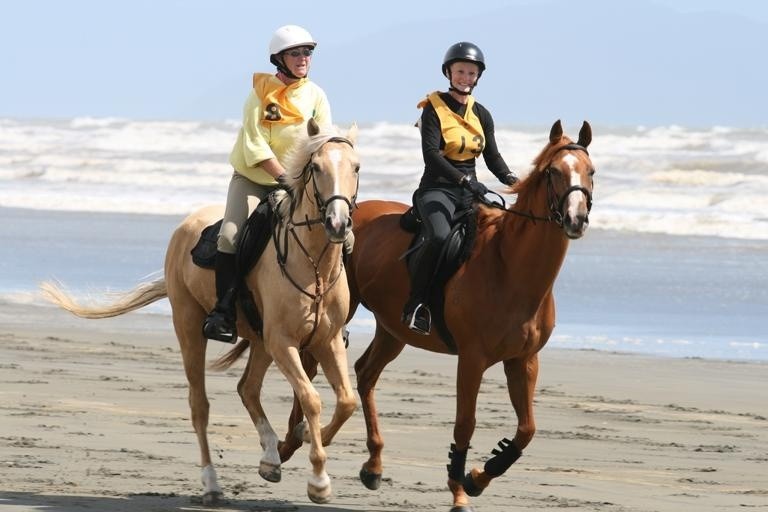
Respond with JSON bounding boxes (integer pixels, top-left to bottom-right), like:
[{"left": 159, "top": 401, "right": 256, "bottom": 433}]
[
  {"left": 348, "top": 118, "right": 597, "bottom": 510},
  {"left": 38, "top": 122, "right": 361, "bottom": 510}
]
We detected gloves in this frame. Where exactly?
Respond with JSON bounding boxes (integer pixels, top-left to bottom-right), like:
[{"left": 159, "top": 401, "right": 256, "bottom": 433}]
[
  {"left": 463, "top": 176, "right": 488, "bottom": 198},
  {"left": 504, "top": 172, "right": 520, "bottom": 187},
  {"left": 276, "top": 174, "right": 297, "bottom": 205}
]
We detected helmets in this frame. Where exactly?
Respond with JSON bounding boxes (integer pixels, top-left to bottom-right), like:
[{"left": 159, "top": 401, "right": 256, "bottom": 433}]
[
  {"left": 269, "top": 25, "right": 316, "bottom": 64},
  {"left": 442, "top": 41, "right": 485, "bottom": 77}
]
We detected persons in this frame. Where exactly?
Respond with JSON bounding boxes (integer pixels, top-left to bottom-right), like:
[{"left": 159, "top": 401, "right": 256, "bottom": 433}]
[
  {"left": 400, "top": 40, "right": 522, "bottom": 335},
  {"left": 203, "top": 23, "right": 355, "bottom": 335}
]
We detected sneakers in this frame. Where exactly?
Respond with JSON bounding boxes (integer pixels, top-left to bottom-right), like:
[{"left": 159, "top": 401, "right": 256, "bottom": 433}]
[
  {"left": 204, "top": 303, "right": 238, "bottom": 338},
  {"left": 401, "top": 306, "right": 429, "bottom": 333}
]
